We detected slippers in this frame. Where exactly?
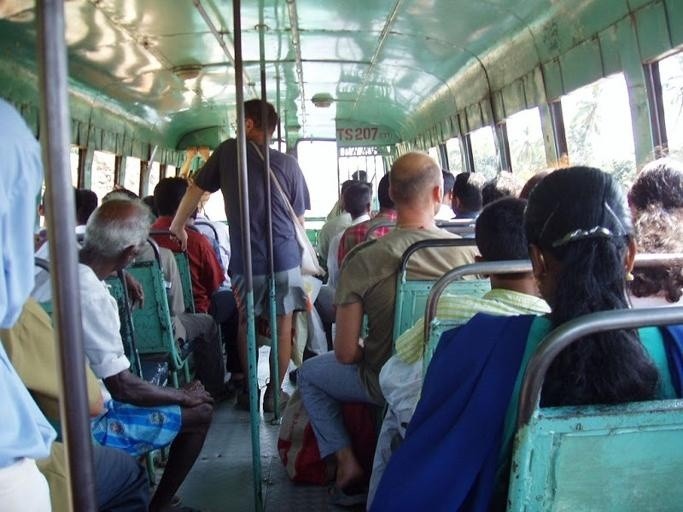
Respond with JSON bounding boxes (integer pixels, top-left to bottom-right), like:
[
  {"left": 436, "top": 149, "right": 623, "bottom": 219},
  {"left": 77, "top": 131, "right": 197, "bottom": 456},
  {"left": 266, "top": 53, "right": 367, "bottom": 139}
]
[{"left": 326, "top": 484, "right": 366, "bottom": 506}]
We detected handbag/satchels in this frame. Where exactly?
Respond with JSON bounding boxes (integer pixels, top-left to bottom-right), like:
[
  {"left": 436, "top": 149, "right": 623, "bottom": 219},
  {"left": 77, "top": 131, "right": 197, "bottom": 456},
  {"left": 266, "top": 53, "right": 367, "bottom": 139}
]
[{"left": 256, "top": 216, "right": 328, "bottom": 366}]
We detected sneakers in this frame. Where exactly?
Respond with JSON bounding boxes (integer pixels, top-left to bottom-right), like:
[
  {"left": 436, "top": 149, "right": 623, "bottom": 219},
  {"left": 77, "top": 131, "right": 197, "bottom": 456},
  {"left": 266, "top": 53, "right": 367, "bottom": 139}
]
[
  {"left": 263, "top": 383, "right": 289, "bottom": 412},
  {"left": 289, "top": 368, "right": 298, "bottom": 381},
  {"left": 238, "top": 388, "right": 261, "bottom": 411}
]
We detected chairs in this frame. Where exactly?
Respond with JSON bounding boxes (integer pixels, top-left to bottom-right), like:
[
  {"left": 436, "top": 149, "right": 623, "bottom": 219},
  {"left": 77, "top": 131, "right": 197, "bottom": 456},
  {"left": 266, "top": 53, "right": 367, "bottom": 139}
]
[
  {"left": 359, "top": 220, "right": 683, "bottom": 512},
  {"left": 34, "top": 223, "right": 221, "bottom": 485}
]
